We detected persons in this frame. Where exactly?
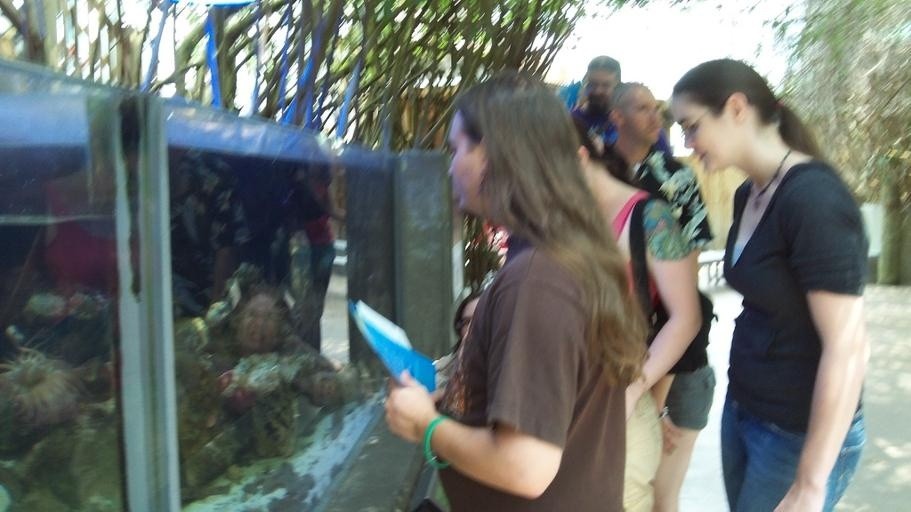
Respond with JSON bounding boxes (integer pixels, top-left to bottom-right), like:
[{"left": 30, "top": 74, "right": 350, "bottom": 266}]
[
  {"left": 557, "top": 54, "right": 675, "bottom": 161},
  {"left": 451, "top": 286, "right": 490, "bottom": 348},
  {"left": 596, "top": 139, "right": 720, "bottom": 512},
  {"left": 1, "top": 137, "right": 344, "bottom": 491},
  {"left": 561, "top": 93, "right": 709, "bottom": 512},
  {"left": 381, "top": 72, "right": 655, "bottom": 511},
  {"left": 670, "top": 56, "right": 872, "bottom": 511},
  {"left": 597, "top": 80, "right": 720, "bottom": 258}
]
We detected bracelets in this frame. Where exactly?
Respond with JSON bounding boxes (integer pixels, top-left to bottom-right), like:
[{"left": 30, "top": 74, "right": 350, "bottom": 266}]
[
  {"left": 657, "top": 405, "right": 670, "bottom": 420},
  {"left": 422, "top": 414, "right": 453, "bottom": 471}
]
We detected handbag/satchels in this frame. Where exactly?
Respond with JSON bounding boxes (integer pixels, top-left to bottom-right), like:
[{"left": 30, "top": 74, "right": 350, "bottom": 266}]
[{"left": 647, "top": 291, "right": 720, "bottom": 376}]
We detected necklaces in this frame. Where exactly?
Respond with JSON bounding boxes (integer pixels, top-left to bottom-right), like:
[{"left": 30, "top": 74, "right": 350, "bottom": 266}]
[{"left": 749, "top": 147, "right": 795, "bottom": 212}]
